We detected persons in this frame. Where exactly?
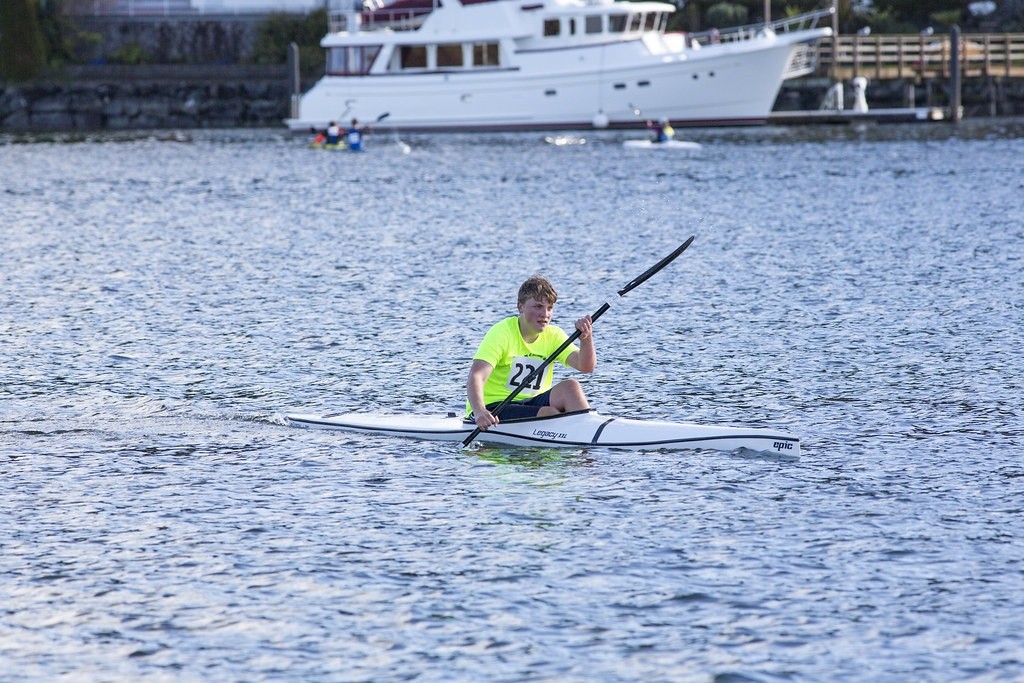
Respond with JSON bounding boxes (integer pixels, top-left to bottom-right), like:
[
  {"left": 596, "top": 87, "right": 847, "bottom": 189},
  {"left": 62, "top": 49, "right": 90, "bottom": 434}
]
[
  {"left": 647, "top": 116, "right": 676, "bottom": 144},
  {"left": 821, "top": 77, "right": 845, "bottom": 112},
  {"left": 314, "top": 120, "right": 347, "bottom": 147},
  {"left": 467, "top": 277, "right": 596, "bottom": 433},
  {"left": 347, "top": 118, "right": 372, "bottom": 152}
]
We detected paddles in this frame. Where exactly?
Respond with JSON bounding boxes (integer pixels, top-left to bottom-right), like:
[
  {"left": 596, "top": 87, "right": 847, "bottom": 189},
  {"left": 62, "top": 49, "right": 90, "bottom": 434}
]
[
  {"left": 453, "top": 235, "right": 695, "bottom": 449},
  {"left": 373, "top": 112, "right": 391, "bottom": 124}
]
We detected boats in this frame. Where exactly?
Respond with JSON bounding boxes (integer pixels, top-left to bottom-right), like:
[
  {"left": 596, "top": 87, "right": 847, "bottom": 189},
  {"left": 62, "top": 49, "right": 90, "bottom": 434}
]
[
  {"left": 309, "top": 134, "right": 349, "bottom": 151},
  {"left": 285, "top": 401, "right": 803, "bottom": 466},
  {"left": 285, "top": 0, "right": 836, "bottom": 141},
  {"left": 619, "top": 139, "right": 703, "bottom": 152}
]
